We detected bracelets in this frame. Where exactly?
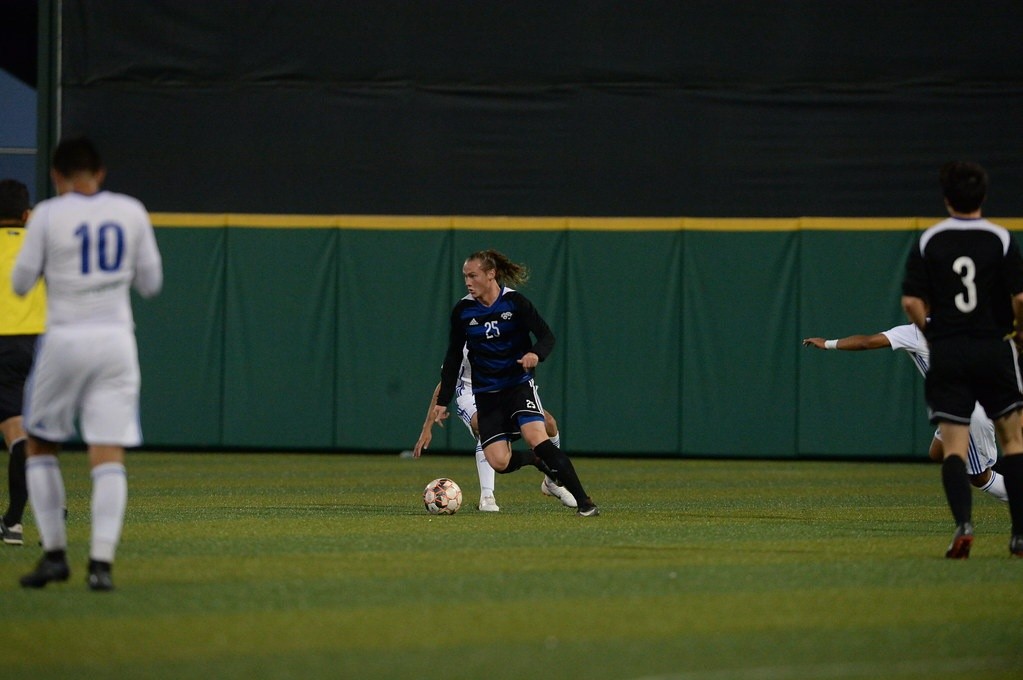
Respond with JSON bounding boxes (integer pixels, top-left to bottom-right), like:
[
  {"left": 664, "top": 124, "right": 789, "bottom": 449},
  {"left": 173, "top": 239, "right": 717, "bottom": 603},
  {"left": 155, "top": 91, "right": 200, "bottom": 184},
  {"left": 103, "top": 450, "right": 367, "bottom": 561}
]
[{"left": 825, "top": 339, "right": 838, "bottom": 350}]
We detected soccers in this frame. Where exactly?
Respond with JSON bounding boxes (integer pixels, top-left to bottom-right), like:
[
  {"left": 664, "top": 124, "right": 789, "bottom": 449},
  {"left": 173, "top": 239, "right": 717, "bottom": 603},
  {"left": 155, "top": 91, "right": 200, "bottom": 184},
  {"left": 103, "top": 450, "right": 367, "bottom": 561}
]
[{"left": 423, "top": 478, "right": 463, "bottom": 515}]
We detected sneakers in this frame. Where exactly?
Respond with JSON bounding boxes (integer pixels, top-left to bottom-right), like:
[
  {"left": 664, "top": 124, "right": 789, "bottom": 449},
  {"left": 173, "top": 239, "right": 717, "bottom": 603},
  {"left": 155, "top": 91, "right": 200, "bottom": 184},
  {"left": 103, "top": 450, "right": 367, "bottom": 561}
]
[
  {"left": 577, "top": 497, "right": 599, "bottom": 517},
  {"left": 479, "top": 495, "right": 499, "bottom": 511},
  {"left": 0, "top": 516, "right": 23, "bottom": 545},
  {"left": 530, "top": 448, "right": 562, "bottom": 487},
  {"left": 1009, "top": 532, "right": 1023, "bottom": 558},
  {"left": 20, "top": 550, "right": 70, "bottom": 588},
  {"left": 88, "top": 559, "right": 113, "bottom": 591},
  {"left": 542, "top": 474, "right": 578, "bottom": 508},
  {"left": 946, "top": 523, "right": 973, "bottom": 559}
]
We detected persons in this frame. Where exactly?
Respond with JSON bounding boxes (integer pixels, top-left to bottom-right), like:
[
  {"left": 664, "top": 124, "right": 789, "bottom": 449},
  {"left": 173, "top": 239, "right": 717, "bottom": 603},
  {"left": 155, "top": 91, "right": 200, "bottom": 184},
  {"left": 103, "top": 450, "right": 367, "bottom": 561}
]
[
  {"left": 803, "top": 300, "right": 1023, "bottom": 503},
  {"left": 10, "top": 137, "right": 164, "bottom": 589},
  {"left": 899, "top": 161, "right": 1023, "bottom": 559},
  {"left": 433, "top": 249, "right": 600, "bottom": 516},
  {"left": 412, "top": 343, "right": 578, "bottom": 511},
  {"left": 0, "top": 175, "right": 69, "bottom": 546}
]
